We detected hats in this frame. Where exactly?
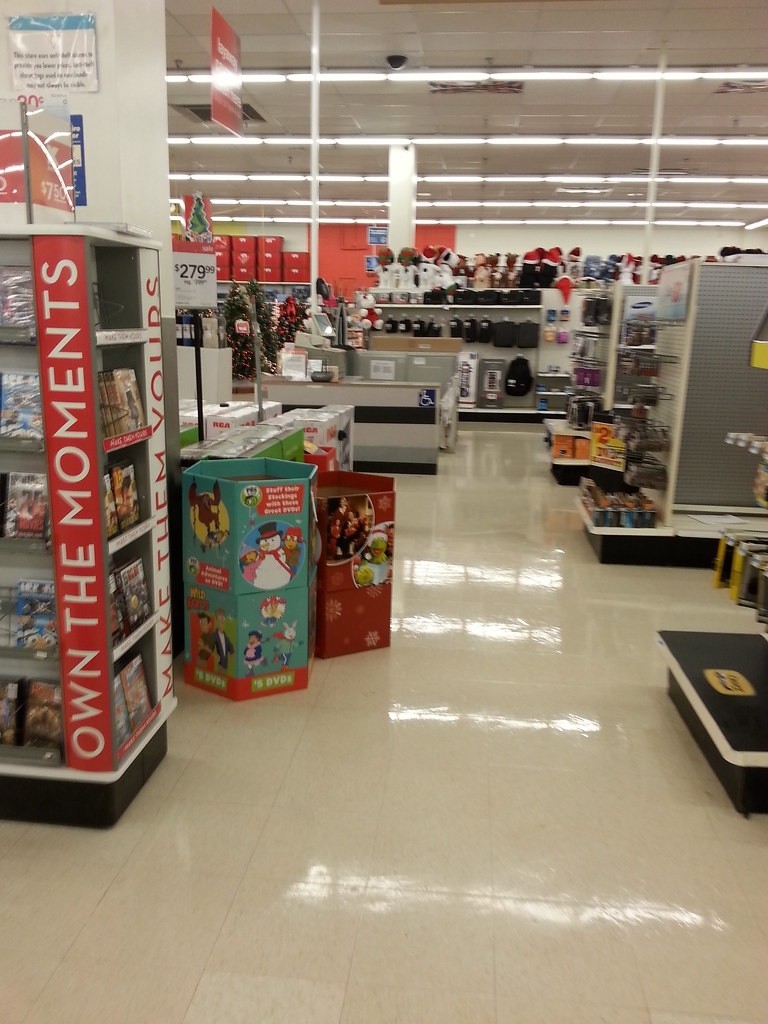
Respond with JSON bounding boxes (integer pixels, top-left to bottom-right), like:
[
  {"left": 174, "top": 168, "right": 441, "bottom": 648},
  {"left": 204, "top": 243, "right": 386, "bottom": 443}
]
[{"left": 373, "top": 243, "right": 579, "bottom": 308}]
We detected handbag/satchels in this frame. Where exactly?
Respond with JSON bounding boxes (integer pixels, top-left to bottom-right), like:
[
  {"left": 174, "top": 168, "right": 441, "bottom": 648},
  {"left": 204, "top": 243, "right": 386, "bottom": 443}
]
[{"left": 387, "top": 289, "right": 541, "bottom": 396}]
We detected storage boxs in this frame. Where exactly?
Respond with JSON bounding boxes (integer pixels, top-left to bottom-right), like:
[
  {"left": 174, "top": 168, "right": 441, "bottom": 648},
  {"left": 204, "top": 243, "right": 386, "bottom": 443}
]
[
  {"left": 172, "top": 233, "right": 310, "bottom": 282},
  {"left": 374, "top": 336, "right": 463, "bottom": 351},
  {"left": 176, "top": 397, "right": 354, "bottom": 470},
  {"left": 536, "top": 373, "right": 572, "bottom": 411}
]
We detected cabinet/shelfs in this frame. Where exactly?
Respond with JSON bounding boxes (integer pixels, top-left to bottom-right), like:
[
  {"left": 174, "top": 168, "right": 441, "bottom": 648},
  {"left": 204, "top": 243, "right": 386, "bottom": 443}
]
[{"left": 0, "top": 220, "right": 177, "bottom": 784}]
